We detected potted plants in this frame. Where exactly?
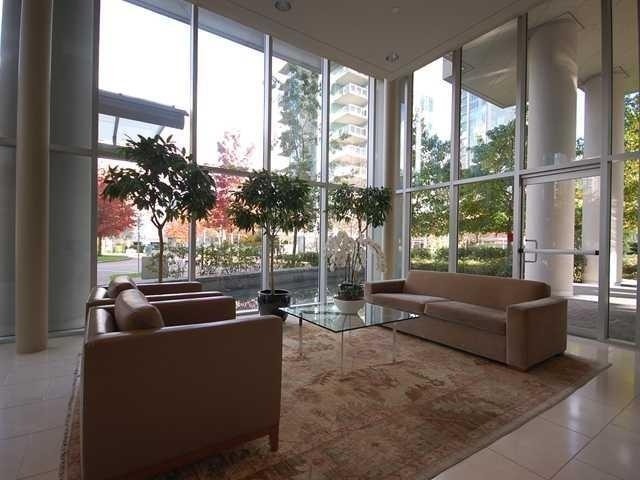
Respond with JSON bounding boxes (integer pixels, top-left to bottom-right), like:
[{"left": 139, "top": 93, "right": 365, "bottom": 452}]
[
  {"left": 320, "top": 182, "right": 394, "bottom": 301},
  {"left": 229, "top": 170, "right": 315, "bottom": 323}
]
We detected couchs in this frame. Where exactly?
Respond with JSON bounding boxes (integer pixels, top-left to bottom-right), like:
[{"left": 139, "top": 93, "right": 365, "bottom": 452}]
[{"left": 364, "top": 269, "right": 568, "bottom": 372}]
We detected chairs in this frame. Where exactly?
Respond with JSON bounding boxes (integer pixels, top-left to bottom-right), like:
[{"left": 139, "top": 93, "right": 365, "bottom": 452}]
[
  {"left": 77, "top": 296, "right": 282, "bottom": 477},
  {"left": 84, "top": 275, "right": 221, "bottom": 321}
]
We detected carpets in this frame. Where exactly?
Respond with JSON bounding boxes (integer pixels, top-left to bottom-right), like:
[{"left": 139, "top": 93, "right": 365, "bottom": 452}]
[{"left": 58, "top": 316, "right": 612, "bottom": 480}]
